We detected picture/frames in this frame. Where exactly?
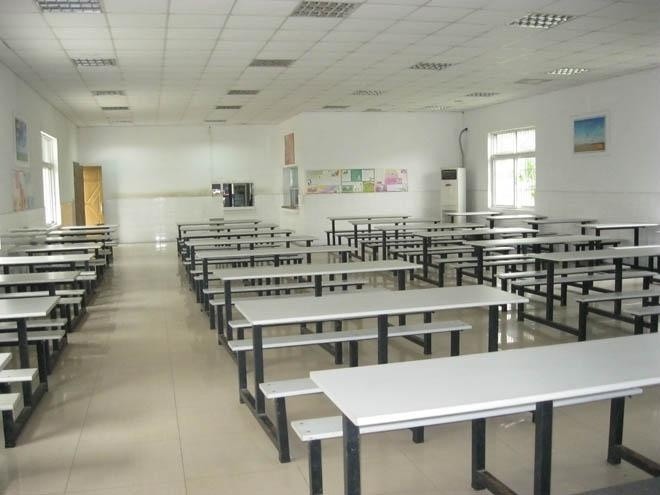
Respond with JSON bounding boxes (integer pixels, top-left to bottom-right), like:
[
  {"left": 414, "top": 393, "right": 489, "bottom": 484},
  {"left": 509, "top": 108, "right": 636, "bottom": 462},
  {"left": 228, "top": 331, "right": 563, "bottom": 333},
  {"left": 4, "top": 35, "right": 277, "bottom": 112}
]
[
  {"left": 10, "top": 112, "right": 32, "bottom": 173},
  {"left": 570, "top": 113, "right": 610, "bottom": 157}
]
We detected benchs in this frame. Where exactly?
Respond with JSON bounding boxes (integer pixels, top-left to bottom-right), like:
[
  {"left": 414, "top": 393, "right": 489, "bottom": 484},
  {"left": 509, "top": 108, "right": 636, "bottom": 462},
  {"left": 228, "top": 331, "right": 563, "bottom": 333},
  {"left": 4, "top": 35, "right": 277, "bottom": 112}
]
[
  {"left": 177, "top": 210, "right": 660, "bottom": 495},
  {"left": 0, "top": 225, "right": 117, "bottom": 449}
]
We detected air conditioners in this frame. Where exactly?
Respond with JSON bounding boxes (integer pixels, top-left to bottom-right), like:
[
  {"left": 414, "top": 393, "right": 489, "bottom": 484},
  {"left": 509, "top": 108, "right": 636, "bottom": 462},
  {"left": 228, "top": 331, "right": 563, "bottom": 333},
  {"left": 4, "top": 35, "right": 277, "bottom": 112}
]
[{"left": 439, "top": 168, "right": 466, "bottom": 239}]
